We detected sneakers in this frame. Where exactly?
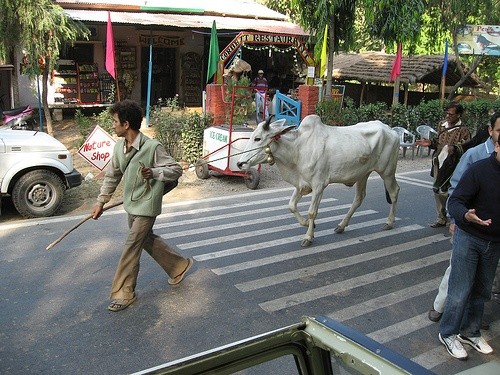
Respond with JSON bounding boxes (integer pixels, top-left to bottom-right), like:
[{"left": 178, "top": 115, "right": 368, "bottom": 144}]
[
  {"left": 457, "top": 333, "right": 494, "bottom": 354},
  {"left": 438, "top": 332, "right": 468, "bottom": 359}
]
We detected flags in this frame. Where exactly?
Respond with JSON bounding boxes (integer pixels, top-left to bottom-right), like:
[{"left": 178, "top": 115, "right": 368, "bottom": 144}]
[
  {"left": 442, "top": 42, "right": 448, "bottom": 75},
  {"left": 106, "top": 11, "right": 117, "bottom": 80},
  {"left": 320, "top": 24, "right": 327, "bottom": 78},
  {"left": 206, "top": 20, "right": 219, "bottom": 83},
  {"left": 390, "top": 41, "right": 402, "bottom": 81}
]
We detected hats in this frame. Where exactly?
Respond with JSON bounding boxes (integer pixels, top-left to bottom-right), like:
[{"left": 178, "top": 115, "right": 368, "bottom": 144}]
[{"left": 258, "top": 69, "right": 264, "bottom": 74}]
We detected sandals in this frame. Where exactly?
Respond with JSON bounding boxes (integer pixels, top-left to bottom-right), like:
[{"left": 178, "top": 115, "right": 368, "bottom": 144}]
[
  {"left": 167, "top": 259, "right": 192, "bottom": 286},
  {"left": 108, "top": 293, "right": 136, "bottom": 311}
]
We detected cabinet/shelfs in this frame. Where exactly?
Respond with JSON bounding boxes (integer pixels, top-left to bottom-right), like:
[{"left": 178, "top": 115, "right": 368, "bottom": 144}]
[
  {"left": 104, "top": 45, "right": 138, "bottom": 72},
  {"left": 55, "top": 59, "right": 80, "bottom": 104},
  {"left": 98, "top": 70, "right": 116, "bottom": 104},
  {"left": 76, "top": 62, "right": 101, "bottom": 104},
  {"left": 181, "top": 51, "right": 203, "bottom": 106}
]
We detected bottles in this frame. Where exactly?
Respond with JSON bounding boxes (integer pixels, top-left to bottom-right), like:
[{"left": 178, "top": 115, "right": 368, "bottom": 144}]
[{"left": 101, "top": 73, "right": 112, "bottom": 102}]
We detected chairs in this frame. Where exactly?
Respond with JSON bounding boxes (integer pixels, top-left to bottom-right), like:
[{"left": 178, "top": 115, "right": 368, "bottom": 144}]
[
  {"left": 416, "top": 125, "right": 438, "bottom": 156},
  {"left": 392, "top": 126, "right": 415, "bottom": 161}
]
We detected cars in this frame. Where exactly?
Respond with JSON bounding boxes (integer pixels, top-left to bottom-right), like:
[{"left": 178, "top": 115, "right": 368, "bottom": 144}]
[{"left": 0, "top": 129, "right": 85, "bottom": 219}]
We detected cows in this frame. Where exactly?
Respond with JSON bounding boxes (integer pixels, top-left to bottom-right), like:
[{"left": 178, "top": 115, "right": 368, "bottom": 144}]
[{"left": 234, "top": 110, "right": 402, "bottom": 248}]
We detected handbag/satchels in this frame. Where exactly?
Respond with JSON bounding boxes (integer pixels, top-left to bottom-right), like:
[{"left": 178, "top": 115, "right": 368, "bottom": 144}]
[{"left": 162, "top": 181, "right": 179, "bottom": 195}]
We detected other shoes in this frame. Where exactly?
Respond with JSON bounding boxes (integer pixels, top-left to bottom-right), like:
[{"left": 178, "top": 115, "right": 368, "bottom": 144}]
[
  {"left": 429, "top": 308, "right": 441, "bottom": 322},
  {"left": 431, "top": 222, "right": 446, "bottom": 228}
]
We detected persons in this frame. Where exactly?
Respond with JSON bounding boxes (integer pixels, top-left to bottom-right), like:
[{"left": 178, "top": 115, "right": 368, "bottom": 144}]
[
  {"left": 416, "top": 103, "right": 471, "bottom": 228},
  {"left": 91, "top": 100, "right": 193, "bottom": 311},
  {"left": 251, "top": 70, "right": 269, "bottom": 123},
  {"left": 428, "top": 112, "right": 500, "bottom": 358}
]
